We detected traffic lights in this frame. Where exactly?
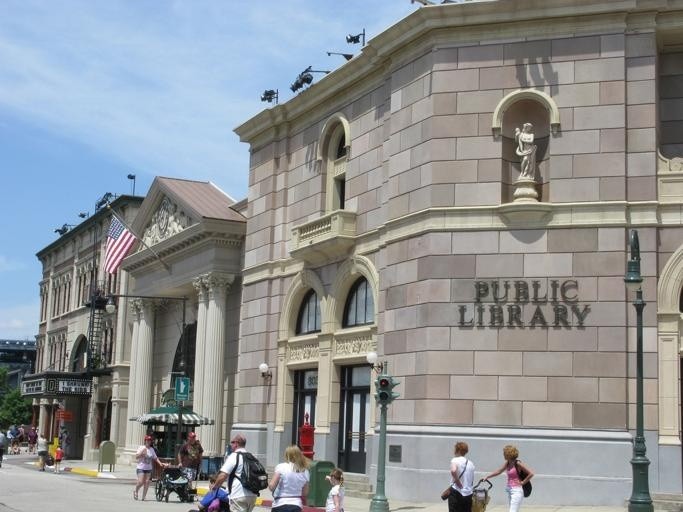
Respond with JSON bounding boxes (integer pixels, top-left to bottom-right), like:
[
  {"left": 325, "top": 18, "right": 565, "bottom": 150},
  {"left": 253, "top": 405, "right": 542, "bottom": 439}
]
[{"left": 372, "top": 374, "right": 401, "bottom": 405}]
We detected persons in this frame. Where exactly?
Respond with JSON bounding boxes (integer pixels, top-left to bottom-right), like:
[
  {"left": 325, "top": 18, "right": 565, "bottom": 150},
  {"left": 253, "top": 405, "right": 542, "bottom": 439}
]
[
  {"left": 324, "top": 468, "right": 344, "bottom": 512},
  {"left": 482, "top": 445, "right": 533, "bottom": 512},
  {"left": 198, "top": 479, "right": 229, "bottom": 512},
  {"left": 178, "top": 432, "right": 203, "bottom": 489},
  {"left": 133, "top": 434, "right": 164, "bottom": 501},
  {"left": 0, "top": 424, "right": 62, "bottom": 474},
  {"left": 449, "top": 442, "right": 476, "bottom": 512},
  {"left": 513, "top": 123, "right": 537, "bottom": 181},
  {"left": 269, "top": 445, "right": 310, "bottom": 512},
  {"left": 213, "top": 435, "right": 257, "bottom": 512}
]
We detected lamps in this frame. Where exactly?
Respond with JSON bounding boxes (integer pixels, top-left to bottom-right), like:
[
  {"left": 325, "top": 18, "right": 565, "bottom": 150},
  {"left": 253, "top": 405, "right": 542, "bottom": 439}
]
[
  {"left": 258, "top": 27, "right": 366, "bottom": 106},
  {"left": 364, "top": 349, "right": 385, "bottom": 375},
  {"left": 51, "top": 172, "right": 136, "bottom": 238},
  {"left": 256, "top": 361, "right": 273, "bottom": 381}
]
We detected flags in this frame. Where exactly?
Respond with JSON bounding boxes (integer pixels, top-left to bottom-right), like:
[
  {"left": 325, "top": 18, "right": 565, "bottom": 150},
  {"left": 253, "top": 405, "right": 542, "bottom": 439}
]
[{"left": 102, "top": 214, "right": 135, "bottom": 275}]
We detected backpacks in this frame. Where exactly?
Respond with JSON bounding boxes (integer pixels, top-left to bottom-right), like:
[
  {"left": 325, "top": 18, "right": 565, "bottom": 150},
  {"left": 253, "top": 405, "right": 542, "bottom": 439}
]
[
  {"left": 507, "top": 459, "right": 532, "bottom": 497},
  {"left": 230, "top": 450, "right": 269, "bottom": 493}
]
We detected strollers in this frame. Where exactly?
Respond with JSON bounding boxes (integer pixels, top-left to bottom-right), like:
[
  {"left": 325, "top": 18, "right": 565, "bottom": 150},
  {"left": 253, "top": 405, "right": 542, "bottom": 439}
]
[
  {"left": 152, "top": 458, "right": 196, "bottom": 503},
  {"left": 9, "top": 437, "right": 24, "bottom": 455},
  {"left": 470, "top": 477, "right": 495, "bottom": 512}
]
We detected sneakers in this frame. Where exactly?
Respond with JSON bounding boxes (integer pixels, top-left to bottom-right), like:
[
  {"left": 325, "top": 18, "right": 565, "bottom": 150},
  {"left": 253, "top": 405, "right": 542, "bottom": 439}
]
[
  {"left": 134, "top": 491, "right": 138, "bottom": 500},
  {"left": 142, "top": 494, "right": 146, "bottom": 500}
]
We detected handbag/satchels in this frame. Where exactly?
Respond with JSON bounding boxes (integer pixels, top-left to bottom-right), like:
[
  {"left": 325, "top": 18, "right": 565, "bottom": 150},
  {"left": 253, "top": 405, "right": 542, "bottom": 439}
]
[{"left": 440, "top": 487, "right": 453, "bottom": 500}]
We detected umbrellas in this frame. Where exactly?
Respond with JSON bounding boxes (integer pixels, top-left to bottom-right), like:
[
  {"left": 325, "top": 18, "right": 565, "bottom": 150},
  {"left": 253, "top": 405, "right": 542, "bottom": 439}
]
[{"left": 129, "top": 405, "right": 215, "bottom": 458}]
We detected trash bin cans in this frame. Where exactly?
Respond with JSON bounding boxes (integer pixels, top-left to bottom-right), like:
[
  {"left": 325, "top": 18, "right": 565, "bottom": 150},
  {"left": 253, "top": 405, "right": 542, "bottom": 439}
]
[
  {"left": 306, "top": 460, "right": 336, "bottom": 508},
  {"left": 97, "top": 440, "right": 116, "bottom": 474}
]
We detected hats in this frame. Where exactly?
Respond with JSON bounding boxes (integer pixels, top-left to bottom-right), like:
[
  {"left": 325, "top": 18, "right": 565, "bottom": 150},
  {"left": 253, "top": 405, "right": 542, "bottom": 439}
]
[{"left": 142, "top": 431, "right": 197, "bottom": 440}]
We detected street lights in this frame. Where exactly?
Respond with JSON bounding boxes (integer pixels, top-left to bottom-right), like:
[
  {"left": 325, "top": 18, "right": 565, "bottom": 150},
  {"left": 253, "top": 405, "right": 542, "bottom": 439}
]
[
  {"left": 103, "top": 292, "right": 190, "bottom": 462},
  {"left": 619, "top": 227, "right": 656, "bottom": 512}
]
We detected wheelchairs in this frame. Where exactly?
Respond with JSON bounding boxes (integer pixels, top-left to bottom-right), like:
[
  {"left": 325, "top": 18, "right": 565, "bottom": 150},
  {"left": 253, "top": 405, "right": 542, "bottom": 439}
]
[{"left": 184, "top": 479, "right": 233, "bottom": 512}]
